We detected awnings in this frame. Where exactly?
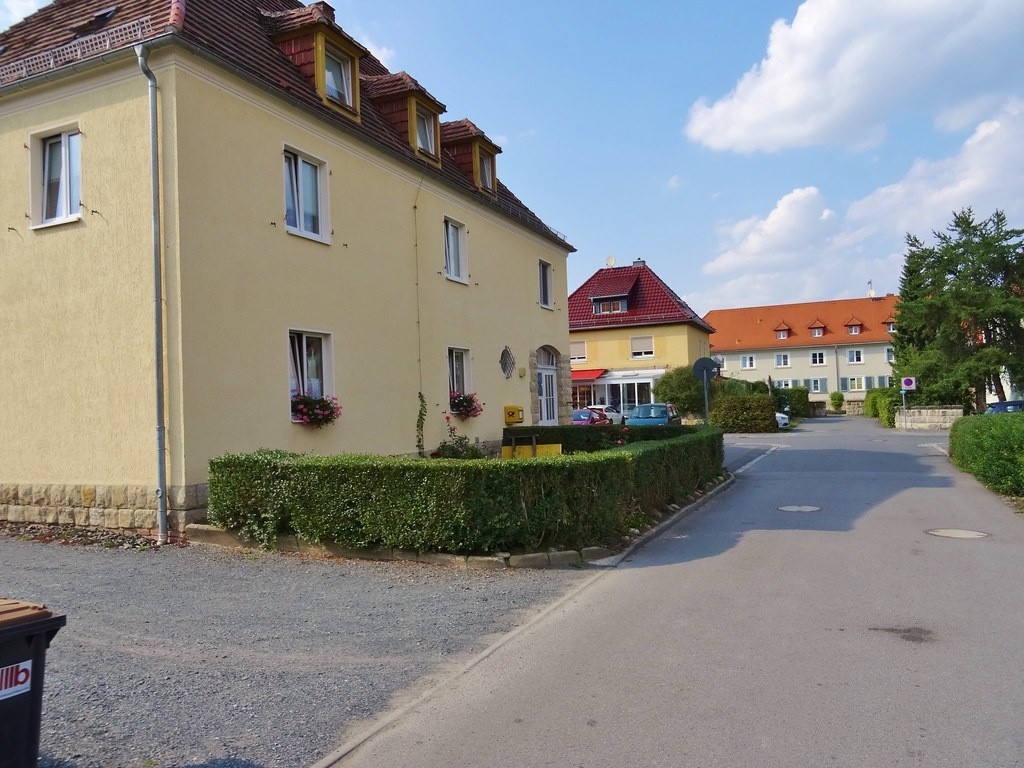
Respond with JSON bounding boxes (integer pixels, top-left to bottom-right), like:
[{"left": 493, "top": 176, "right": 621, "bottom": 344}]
[{"left": 571, "top": 368, "right": 606, "bottom": 382}]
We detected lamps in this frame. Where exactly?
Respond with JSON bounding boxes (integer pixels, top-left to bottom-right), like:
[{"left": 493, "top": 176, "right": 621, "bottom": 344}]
[{"left": 519, "top": 367, "right": 526, "bottom": 377}]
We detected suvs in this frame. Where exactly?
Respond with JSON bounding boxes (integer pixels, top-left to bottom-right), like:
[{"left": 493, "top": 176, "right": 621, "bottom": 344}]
[
  {"left": 623, "top": 401, "right": 683, "bottom": 427},
  {"left": 982, "top": 400, "right": 1024, "bottom": 414}
]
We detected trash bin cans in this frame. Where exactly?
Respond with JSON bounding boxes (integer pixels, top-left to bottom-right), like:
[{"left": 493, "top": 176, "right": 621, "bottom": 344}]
[{"left": 0, "top": 596, "right": 67, "bottom": 768}]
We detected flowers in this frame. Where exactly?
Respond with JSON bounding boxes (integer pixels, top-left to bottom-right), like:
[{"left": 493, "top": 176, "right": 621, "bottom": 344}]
[
  {"left": 450, "top": 391, "right": 486, "bottom": 421},
  {"left": 291, "top": 393, "right": 343, "bottom": 431}
]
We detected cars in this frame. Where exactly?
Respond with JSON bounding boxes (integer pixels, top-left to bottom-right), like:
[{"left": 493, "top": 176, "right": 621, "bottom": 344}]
[
  {"left": 573, "top": 408, "right": 613, "bottom": 426},
  {"left": 580, "top": 405, "right": 625, "bottom": 425},
  {"left": 617, "top": 404, "right": 636, "bottom": 419},
  {"left": 773, "top": 411, "right": 790, "bottom": 430}
]
